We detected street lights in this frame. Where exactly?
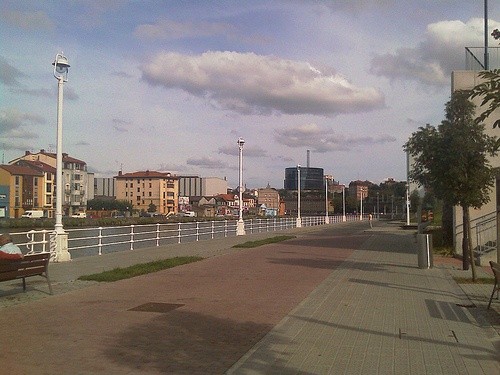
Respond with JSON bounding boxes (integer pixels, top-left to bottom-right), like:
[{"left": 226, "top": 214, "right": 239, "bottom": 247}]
[
  {"left": 325, "top": 175, "right": 330, "bottom": 224},
  {"left": 45, "top": 47, "right": 73, "bottom": 262},
  {"left": 235, "top": 136, "right": 248, "bottom": 236},
  {"left": 360, "top": 187, "right": 363, "bottom": 220},
  {"left": 343, "top": 184, "right": 346, "bottom": 222},
  {"left": 295, "top": 163, "right": 302, "bottom": 227}
]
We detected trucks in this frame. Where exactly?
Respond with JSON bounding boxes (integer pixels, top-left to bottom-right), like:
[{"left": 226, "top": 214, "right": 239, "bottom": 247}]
[
  {"left": 72, "top": 213, "right": 86, "bottom": 218},
  {"left": 21, "top": 210, "right": 44, "bottom": 219},
  {"left": 184, "top": 211, "right": 195, "bottom": 217}
]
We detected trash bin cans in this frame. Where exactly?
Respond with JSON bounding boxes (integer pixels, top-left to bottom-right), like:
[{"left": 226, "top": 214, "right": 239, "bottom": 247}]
[{"left": 418, "top": 234, "right": 432, "bottom": 269}]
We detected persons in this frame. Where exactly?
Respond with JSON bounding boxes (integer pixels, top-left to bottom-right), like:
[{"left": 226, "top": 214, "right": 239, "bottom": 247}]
[{"left": 0, "top": 233, "right": 24, "bottom": 260}]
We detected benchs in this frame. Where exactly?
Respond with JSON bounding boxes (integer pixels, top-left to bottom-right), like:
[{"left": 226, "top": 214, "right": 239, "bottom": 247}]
[
  {"left": 0, "top": 253, "right": 53, "bottom": 296},
  {"left": 487, "top": 260, "right": 500, "bottom": 309}
]
